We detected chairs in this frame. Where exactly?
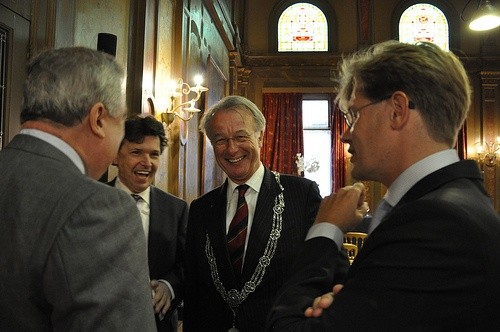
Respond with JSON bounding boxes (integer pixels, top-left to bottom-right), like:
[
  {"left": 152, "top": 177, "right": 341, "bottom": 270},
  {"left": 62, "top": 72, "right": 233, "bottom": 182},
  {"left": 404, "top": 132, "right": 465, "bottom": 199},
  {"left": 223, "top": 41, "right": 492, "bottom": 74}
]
[{"left": 343, "top": 231, "right": 368, "bottom": 266}]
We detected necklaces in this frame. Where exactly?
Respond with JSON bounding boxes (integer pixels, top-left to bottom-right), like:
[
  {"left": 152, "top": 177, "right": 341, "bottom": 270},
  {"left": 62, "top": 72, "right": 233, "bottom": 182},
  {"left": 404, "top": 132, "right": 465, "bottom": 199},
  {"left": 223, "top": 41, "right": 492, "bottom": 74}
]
[{"left": 205, "top": 169, "right": 285, "bottom": 332}]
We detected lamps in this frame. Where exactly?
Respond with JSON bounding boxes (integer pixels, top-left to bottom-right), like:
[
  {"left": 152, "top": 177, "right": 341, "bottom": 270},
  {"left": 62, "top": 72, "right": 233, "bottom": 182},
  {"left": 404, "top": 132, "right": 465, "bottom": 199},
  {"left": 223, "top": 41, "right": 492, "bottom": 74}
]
[
  {"left": 474, "top": 139, "right": 500, "bottom": 167},
  {"left": 161, "top": 73, "right": 208, "bottom": 147}
]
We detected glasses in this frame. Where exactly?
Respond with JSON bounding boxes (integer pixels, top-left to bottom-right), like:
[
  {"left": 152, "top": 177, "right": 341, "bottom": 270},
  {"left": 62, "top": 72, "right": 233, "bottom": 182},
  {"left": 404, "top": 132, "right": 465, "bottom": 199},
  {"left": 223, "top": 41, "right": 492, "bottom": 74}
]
[
  {"left": 343, "top": 95, "right": 415, "bottom": 128},
  {"left": 208, "top": 129, "right": 260, "bottom": 146}
]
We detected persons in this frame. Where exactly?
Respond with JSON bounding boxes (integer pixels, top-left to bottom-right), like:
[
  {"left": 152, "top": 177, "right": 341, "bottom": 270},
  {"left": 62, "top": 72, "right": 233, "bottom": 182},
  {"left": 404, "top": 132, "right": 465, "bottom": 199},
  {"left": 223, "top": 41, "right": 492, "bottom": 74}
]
[
  {"left": 359, "top": 203, "right": 372, "bottom": 234},
  {"left": 181, "top": 95, "right": 322, "bottom": 332},
  {"left": 266, "top": 38, "right": 500, "bottom": 332},
  {"left": 0, "top": 46, "right": 157, "bottom": 332},
  {"left": 102, "top": 114, "right": 188, "bottom": 332}
]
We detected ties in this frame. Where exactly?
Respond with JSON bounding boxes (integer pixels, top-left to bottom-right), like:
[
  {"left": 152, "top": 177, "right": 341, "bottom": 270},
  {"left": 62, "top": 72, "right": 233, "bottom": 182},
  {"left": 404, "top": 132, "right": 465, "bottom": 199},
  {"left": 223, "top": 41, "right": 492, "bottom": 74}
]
[
  {"left": 130, "top": 194, "right": 141, "bottom": 201},
  {"left": 226, "top": 184, "right": 249, "bottom": 280},
  {"left": 368, "top": 199, "right": 390, "bottom": 236}
]
